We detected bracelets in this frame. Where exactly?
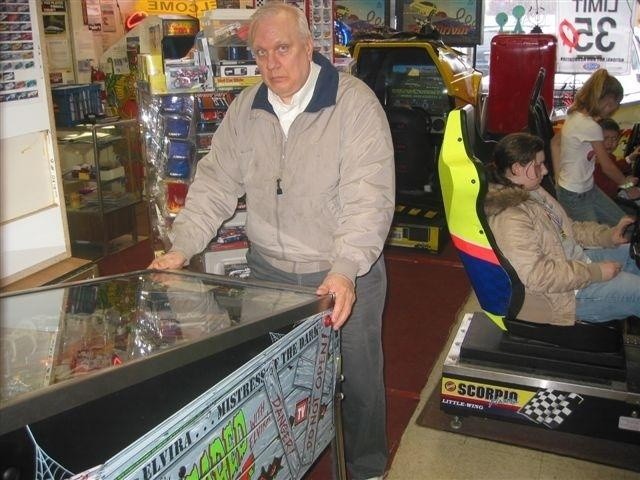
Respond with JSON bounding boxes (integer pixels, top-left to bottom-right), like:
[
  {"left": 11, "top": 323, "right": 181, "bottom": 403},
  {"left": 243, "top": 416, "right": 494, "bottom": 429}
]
[{"left": 625, "top": 156, "right": 632, "bottom": 163}]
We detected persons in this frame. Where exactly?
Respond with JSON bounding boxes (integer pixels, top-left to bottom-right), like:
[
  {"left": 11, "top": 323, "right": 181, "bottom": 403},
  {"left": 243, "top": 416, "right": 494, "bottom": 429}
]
[
  {"left": 145, "top": 2, "right": 396, "bottom": 480},
  {"left": 481, "top": 133, "right": 640, "bottom": 327},
  {"left": 550, "top": 68, "right": 639, "bottom": 227},
  {"left": 592, "top": 118, "right": 640, "bottom": 216}
]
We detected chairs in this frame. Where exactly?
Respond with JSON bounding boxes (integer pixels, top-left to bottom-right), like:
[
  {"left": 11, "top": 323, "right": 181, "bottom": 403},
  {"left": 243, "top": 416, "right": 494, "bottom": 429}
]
[
  {"left": 438, "top": 103, "right": 629, "bottom": 383},
  {"left": 385, "top": 107, "right": 442, "bottom": 212},
  {"left": 528, "top": 67, "right": 556, "bottom": 181}
]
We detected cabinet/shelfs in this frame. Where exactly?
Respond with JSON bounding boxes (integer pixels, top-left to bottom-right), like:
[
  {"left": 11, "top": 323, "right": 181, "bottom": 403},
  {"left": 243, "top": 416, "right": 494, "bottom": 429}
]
[
  {"left": 136, "top": 85, "right": 249, "bottom": 279},
  {"left": 56, "top": 116, "right": 150, "bottom": 261}
]
